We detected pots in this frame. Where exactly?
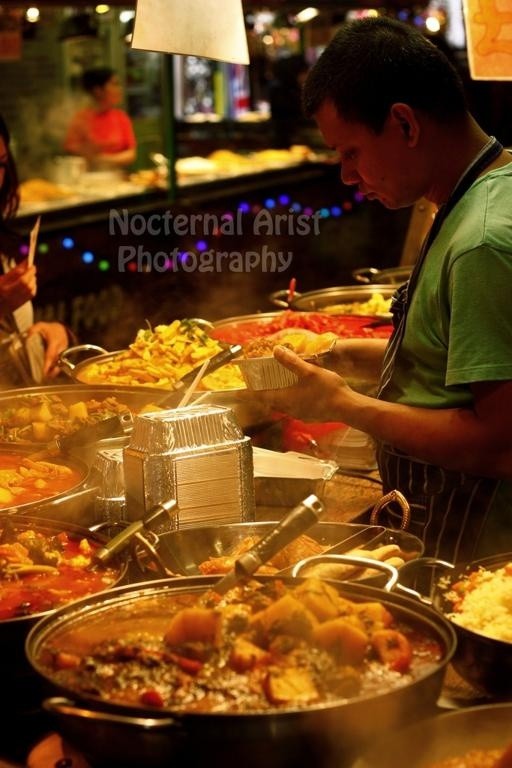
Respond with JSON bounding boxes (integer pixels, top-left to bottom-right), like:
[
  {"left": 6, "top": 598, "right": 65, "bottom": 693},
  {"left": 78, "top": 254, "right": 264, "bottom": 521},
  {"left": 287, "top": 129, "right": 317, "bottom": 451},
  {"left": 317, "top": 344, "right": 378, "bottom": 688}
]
[{"left": 0, "top": 264, "right": 511, "bottom": 768}]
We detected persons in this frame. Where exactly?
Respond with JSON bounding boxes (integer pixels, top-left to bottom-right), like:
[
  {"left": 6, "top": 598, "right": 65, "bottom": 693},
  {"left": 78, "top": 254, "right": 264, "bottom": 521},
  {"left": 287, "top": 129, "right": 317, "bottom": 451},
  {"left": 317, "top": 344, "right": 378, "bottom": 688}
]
[
  {"left": 63, "top": 65, "right": 138, "bottom": 171},
  {"left": 1, "top": 117, "right": 79, "bottom": 384},
  {"left": 233, "top": 16, "right": 512, "bottom": 712}
]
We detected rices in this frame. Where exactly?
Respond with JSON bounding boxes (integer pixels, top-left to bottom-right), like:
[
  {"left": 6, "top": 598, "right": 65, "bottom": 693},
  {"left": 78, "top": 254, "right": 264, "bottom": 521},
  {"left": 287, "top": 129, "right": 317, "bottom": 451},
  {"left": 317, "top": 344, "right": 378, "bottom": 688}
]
[{"left": 438, "top": 565, "right": 512, "bottom": 642}]
